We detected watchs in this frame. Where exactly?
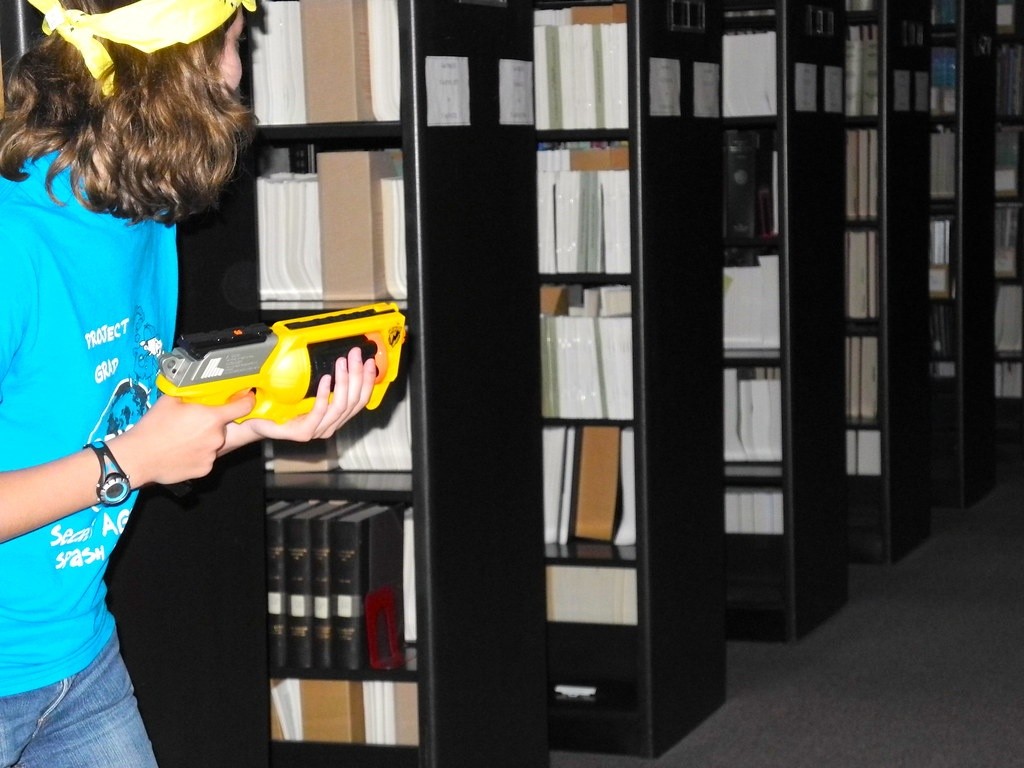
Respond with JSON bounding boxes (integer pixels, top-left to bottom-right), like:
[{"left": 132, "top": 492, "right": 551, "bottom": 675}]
[{"left": 82, "top": 440, "right": 132, "bottom": 506}]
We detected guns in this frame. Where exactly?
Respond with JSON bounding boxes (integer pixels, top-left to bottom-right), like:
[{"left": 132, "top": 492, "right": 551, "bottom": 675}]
[{"left": 152, "top": 298, "right": 409, "bottom": 426}]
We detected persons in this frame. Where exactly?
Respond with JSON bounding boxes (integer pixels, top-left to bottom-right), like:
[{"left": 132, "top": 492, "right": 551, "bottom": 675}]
[{"left": 0, "top": 0, "right": 377, "bottom": 767}]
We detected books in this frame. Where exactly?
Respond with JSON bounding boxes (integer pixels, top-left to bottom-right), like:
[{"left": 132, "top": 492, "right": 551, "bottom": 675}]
[
  {"left": 993, "top": 125, "right": 1018, "bottom": 198},
  {"left": 996, "top": 285, "right": 1023, "bottom": 352},
  {"left": 845, "top": 336, "right": 878, "bottom": 423},
  {"left": 261, "top": 370, "right": 413, "bottom": 472},
  {"left": 994, "top": 202, "right": 1018, "bottom": 279},
  {"left": 843, "top": 0, "right": 880, "bottom": 117},
  {"left": 847, "top": 430, "right": 882, "bottom": 538},
  {"left": 539, "top": 284, "right": 633, "bottom": 420},
  {"left": 848, "top": 128, "right": 878, "bottom": 222},
  {"left": 716, "top": 9, "right": 778, "bottom": 117},
  {"left": 994, "top": 360, "right": 1023, "bottom": 399},
  {"left": 543, "top": 427, "right": 637, "bottom": 562},
  {"left": 929, "top": 219, "right": 951, "bottom": 300},
  {"left": 537, "top": 141, "right": 627, "bottom": 274},
  {"left": 722, "top": 254, "right": 780, "bottom": 350},
  {"left": 928, "top": 305, "right": 958, "bottom": 378},
  {"left": 544, "top": 566, "right": 640, "bottom": 697},
  {"left": 994, "top": 1, "right": 1022, "bottom": 118},
  {"left": 930, "top": 46, "right": 956, "bottom": 116},
  {"left": 931, "top": 124, "right": 957, "bottom": 199},
  {"left": 245, "top": 0, "right": 407, "bottom": 125},
  {"left": 724, "top": 367, "right": 784, "bottom": 463},
  {"left": 249, "top": 145, "right": 408, "bottom": 312},
  {"left": 929, "top": 0, "right": 955, "bottom": 26},
  {"left": 532, "top": 3, "right": 632, "bottom": 130},
  {"left": 262, "top": 490, "right": 418, "bottom": 748},
  {"left": 720, "top": 128, "right": 781, "bottom": 242},
  {"left": 724, "top": 486, "right": 785, "bottom": 614},
  {"left": 845, "top": 230, "right": 879, "bottom": 320}
]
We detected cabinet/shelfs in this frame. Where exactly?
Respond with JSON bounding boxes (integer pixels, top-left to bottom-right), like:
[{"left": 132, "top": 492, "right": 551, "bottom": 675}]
[
  {"left": 930, "top": 0, "right": 1024, "bottom": 508},
  {"left": 845, "top": 0, "right": 932, "bottom": 568},
  {"left": 252, "top": 0, "right": 552, "bottom": 768},
  {"left": 534, "top": 0, "right": 726, "bottom": 761},
  {"left": 722, "top": 0, "right": 848, "bottom": 646}
]
